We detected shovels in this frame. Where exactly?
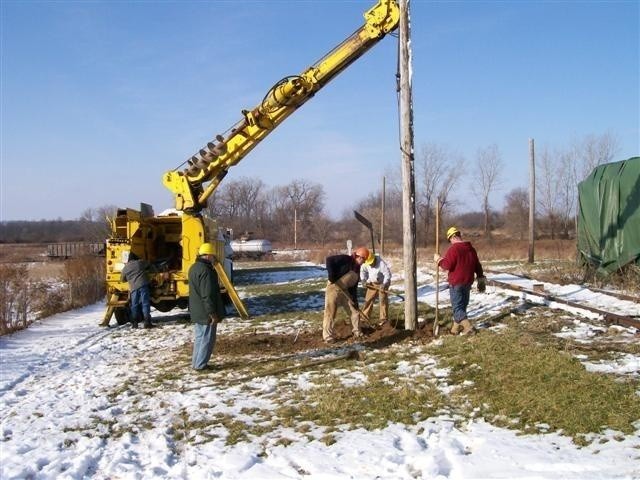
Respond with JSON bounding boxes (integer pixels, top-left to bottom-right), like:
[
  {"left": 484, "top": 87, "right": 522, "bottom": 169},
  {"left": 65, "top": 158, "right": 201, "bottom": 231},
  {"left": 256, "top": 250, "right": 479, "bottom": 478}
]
[
  {"left": 430, "top": 197, "right": 441, "bottom": 337},
  {"left": 333, "top": 280, "right": 382, "bottom": 330}
]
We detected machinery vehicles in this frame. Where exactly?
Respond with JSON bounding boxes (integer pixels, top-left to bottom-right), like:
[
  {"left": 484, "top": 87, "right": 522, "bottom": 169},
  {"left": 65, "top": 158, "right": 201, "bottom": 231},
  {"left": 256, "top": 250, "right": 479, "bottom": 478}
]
[
  {"left": 223, "top": 225, "right": 276, "bottom": 263},
  {"left": 99, "top": 0, "right": 411, "bottom": 334}
]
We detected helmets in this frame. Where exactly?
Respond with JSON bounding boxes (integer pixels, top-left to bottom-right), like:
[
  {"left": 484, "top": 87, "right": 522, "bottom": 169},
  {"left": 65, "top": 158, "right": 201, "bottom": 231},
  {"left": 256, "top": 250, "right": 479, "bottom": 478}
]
[
  {"left": 355, "top": 247, "right": 370, "bottom": 260},
  {"left": 198, "top": 242, "right": 217, "bottom": 260},
  {"left": 364, "top": 252, "right": 377, "bottom": 265},
  {"left": 446, "top": 225, "right": 461, "bottom": 243}
]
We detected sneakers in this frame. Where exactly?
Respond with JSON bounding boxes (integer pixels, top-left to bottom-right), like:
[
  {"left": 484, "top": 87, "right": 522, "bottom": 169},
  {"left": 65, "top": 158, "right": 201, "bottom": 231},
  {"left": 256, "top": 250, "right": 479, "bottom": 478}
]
[
  {"left": 131, "top": 319, "right": 157, "bottom": 329},
  {"left": 191, "top": 363, "right": 217, "bottom": 375}
]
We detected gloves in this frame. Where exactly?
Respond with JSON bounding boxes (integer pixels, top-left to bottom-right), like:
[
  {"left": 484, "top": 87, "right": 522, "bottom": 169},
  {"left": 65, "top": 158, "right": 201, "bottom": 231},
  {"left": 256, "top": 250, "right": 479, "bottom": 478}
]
[
  {"left": 475, "top": 275, "right": 488, "bottom": 293},
  {"left": 433, "top": 252, "right": 444, "bottom": 266}
]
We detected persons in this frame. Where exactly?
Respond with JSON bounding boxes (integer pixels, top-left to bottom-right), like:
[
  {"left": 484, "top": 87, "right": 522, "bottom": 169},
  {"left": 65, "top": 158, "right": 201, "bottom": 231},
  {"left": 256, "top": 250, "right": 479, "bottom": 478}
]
[
  {"left": 322, "top": 245, "right": 370, "bottom": 345},
  {"left": 433, "top": 226, "right": 488, "bottom": 338},
  {"left": 360, "top": 251, "right": 392, "bottom": 329},
  {"left": 187, "top": 243, "right": 228, "bottom": 375},
  {"left": 119, "top": 252, "right": 157, "bottom": 329}
]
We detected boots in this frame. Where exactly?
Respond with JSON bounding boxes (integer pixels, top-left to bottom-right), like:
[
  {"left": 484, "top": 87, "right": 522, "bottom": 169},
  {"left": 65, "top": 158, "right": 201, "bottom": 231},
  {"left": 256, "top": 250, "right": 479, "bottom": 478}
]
[
  {"left": 460, "top": 318, "right": 475, "bottom": 336},
  {"left": 450, "top": 320, "right": 461, "bottom": 334}
]
